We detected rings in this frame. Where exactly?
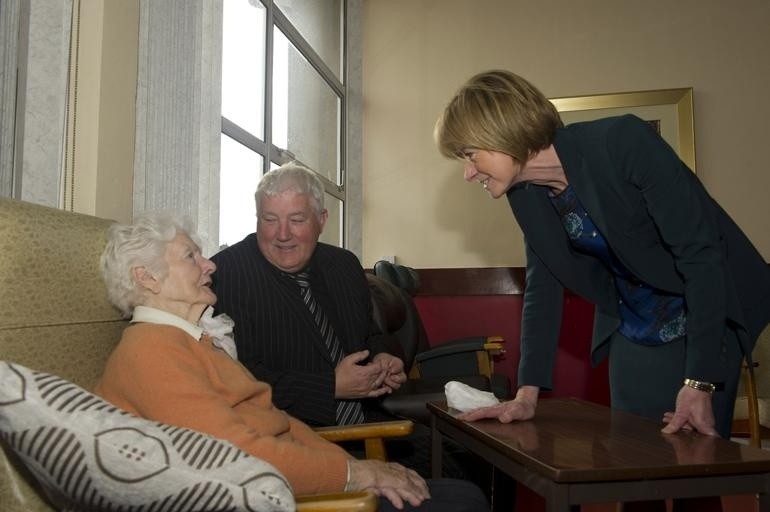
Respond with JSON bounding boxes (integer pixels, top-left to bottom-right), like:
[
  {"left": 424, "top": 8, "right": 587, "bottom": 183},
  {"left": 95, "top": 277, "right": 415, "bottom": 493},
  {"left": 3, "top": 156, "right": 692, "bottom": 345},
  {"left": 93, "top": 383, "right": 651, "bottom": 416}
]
[{"left": 372, "top": 381, "right": 378, "bottom": 390}]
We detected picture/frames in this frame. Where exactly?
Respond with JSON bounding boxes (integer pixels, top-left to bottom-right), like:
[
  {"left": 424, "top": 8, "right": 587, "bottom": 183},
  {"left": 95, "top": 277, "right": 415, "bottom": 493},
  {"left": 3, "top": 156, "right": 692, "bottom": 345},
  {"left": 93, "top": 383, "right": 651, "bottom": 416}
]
[{"left": 546, "top": 85, "right": 697, "bottom": 180}]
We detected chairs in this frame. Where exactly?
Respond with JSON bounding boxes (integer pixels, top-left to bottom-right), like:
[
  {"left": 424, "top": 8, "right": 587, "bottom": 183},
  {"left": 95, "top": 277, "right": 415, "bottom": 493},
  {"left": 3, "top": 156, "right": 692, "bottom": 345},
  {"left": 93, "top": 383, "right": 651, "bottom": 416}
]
[{"left": 2, "top": 201, "right": 507, "bottom": 511}]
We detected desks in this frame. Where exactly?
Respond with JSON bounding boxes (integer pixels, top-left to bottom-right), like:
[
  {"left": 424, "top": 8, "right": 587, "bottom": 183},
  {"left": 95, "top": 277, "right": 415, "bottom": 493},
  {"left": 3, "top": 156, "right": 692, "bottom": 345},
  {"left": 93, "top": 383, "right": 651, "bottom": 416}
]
[{"left": 426, "top": 395, "right": 770, "bottom": 511}]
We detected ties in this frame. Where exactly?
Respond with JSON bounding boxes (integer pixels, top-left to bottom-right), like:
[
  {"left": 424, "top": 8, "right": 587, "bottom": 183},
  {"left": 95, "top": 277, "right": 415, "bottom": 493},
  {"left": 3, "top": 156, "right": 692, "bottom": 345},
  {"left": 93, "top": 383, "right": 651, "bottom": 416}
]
[{"left": 285, "top": 271, "right": 368, "bottom": 427}]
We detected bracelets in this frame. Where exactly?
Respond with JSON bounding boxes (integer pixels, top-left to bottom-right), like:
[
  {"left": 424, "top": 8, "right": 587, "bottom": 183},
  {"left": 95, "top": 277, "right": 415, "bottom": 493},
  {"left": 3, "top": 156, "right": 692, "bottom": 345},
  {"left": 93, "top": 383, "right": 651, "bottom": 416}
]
[
  {"left": 685, "top": 379, "right": 716, "bottom": 393},
  {"left": 344, "top": 460, "right": 351, "bottom": 493}
]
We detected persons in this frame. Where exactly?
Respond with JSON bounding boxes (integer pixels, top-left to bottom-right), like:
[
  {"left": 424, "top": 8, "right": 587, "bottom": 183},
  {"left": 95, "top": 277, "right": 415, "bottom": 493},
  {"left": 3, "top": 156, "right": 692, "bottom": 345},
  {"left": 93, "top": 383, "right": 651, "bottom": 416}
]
[
  {"left": 207, "top": 160, "right": 464, "bottom": 478},
  {"left": 433, "top": 69, "right": 770, "bottom": 512},
  {"left": 95, "top": 219, "right": 493, "bottom": 512}
]
